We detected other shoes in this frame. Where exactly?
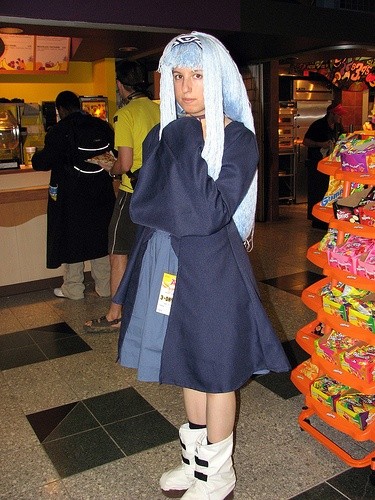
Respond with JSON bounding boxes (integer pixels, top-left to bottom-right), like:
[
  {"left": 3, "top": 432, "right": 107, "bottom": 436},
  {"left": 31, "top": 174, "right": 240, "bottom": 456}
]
[
  {"left": 312, "top": 221, "right": 330, "bottom": 230},
  {"left": 53, "top": 288, "right": 65, "bottom": 297}
]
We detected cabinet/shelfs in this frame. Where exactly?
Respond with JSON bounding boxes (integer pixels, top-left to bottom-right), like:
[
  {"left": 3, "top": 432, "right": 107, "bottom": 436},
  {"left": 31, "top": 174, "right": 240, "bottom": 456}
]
[{"left": 289, "top": 134, "right": 375, "bottom": 467}]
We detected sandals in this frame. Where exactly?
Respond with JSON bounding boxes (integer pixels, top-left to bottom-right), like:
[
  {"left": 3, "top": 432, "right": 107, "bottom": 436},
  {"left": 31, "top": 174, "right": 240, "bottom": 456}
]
[{"left": 83, "top": 316, "right": 121, "bottom": 333}]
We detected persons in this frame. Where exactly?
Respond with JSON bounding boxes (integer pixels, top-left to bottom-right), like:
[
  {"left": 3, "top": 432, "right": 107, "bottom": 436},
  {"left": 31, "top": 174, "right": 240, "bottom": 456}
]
[
  {"left": 32, "top": 90, "right": 111, "bottom": 298},
  {"left": 83, "top": 60, "right": 160, "bottom": 332},
  {"left": 129, "top": 31, "right": 260, "bottom": 500},
  {"left": 303, "top": 103, "right": 348, "bottom": 228}
]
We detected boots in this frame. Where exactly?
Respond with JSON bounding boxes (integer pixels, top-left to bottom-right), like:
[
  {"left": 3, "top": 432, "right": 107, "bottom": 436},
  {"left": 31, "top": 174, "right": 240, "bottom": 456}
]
[
  {"left": 179, "top": 431, "right": 237, "bottom": 500},
  {"left": 159, "top": 423, "right": 207, "bottom": 498}
]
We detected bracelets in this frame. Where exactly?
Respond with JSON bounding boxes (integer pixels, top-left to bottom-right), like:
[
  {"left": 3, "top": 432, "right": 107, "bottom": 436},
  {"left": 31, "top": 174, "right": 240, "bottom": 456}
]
[{"left": 109, "top": 171, "right": 116, "bottom": 178}]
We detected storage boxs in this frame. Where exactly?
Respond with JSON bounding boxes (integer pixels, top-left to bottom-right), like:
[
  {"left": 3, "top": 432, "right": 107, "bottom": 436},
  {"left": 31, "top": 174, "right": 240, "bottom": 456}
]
[{"left": 331, "top": 141, "right": 374, "bottom": 423}]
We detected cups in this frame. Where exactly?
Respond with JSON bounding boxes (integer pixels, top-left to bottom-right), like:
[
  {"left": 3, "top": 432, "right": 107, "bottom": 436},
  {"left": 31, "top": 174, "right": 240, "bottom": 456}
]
[{"left": 25, "top": 146, "right": 36, "bottom": 168}]
